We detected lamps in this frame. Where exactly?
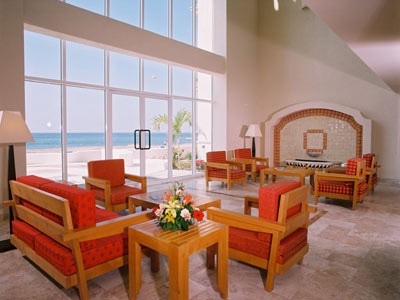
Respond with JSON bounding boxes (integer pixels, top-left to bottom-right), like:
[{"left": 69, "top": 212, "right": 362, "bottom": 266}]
[
  {"left": 0, "top": 111, "right": 35, "bottom": 235},
  {"left": 245, "top": 124, "right": 263, "bottom": 157}
]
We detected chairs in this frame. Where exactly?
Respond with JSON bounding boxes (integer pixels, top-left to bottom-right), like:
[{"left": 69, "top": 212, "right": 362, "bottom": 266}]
[
  {"left": 314, "top": 159, "right": 370, "bottom": 210},
  {"left": 232, "top": 148, "right": 269, "bottom": 182},
  {"left": 363, "top": 154, "right": 378, "bottom": 196},
  {"left": 82, "top": 159, "right": 147, "bottom": 211},
  {"left": 205, "top": 151, "right": 245, "bottom": 190},
  {"left": 206, "top": 181, "right": 317, "bottom": 292}
]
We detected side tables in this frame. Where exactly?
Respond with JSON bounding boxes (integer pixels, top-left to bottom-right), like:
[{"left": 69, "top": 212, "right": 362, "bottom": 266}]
[{"left": 128, "top": 218, "right": 228, "bottom": 300}]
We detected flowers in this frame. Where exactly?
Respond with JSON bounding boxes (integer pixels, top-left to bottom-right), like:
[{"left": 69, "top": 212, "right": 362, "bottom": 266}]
[{"left": 153, "top": 182, "right": 205, "bottom": 231}]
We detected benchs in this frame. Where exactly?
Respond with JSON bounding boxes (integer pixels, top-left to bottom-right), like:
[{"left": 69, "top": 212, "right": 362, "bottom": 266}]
[{"left": 3, "top": 175, "right": 159, "bottom": 300}]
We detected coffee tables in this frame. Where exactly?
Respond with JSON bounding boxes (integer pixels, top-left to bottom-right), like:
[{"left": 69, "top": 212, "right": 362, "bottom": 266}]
[
  {"left": 128, "top": 188, "right": 221, "bottom": 214},
  {"left": 260, "top": 166, "right": 315, "bottom": 195}
]
